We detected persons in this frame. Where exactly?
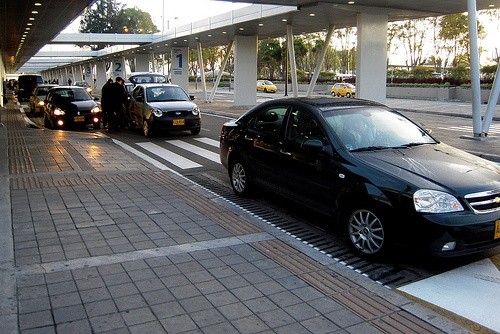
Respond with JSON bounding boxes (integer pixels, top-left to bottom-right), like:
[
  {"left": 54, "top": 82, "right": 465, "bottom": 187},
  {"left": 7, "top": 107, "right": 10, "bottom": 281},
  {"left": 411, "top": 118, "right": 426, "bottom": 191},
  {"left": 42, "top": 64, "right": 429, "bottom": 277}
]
[
  {"left": 3, "top": 81, "right": 6, "bottom": 100},
  {"left": 106, "top": 77, "right": 122, "bottom": 132},
  {"left": 120, "top": 79, "right": 134, "bottom": 126},
  {"left": 68, "top": 78, "right": 72, "bottom": 85},
  {"left": 101, "top": 79, "right": 115, "bottom": 129}
]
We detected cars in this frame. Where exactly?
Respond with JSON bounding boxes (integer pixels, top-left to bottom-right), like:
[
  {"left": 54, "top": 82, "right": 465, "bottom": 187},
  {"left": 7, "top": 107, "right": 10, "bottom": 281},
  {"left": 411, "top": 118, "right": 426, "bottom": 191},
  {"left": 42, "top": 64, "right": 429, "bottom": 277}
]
[
  {"left": 330, "top": 83, "right": 356, "bottom": 98},
  {"left": 72, "top": 81, "right": 92, "bottom": 95},
  {"left": 45, "top": 86, "right": 103, "bottom": 131},
  {"left": 126, "top": 71, "right": 171, "bottom": 84},
  {"left": 257, "top": 79, "right": 277, "bottom": 93},
  {"left": 30, "top": 84, "right": 59, "bottom": 115},
  {"left": 220, "top": 97, "right": 499, "bottom": 262},
  {"left": 129, "top": 83, "right": 201, "bottom": 138}
]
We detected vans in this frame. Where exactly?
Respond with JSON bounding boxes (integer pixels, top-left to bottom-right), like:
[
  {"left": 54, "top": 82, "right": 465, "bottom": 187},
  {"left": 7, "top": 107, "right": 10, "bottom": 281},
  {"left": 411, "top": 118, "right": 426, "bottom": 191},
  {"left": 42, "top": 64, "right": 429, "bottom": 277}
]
[{"left": 17, "top": 75, "right": 44, "bottom": 99}]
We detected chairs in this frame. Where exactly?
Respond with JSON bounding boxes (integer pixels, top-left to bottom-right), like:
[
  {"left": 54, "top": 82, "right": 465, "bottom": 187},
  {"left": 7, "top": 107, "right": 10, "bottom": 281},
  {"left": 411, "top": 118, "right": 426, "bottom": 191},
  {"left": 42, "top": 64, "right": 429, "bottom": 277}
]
[
  {"left": 37, "top": 89, "right": 44, "bottom": 95},
  {"left": 259, "top": 111, "right": 281, "bottom": 134},
  {"left": 68, "top": 91, "right": 76, "bottom": 97}
]
